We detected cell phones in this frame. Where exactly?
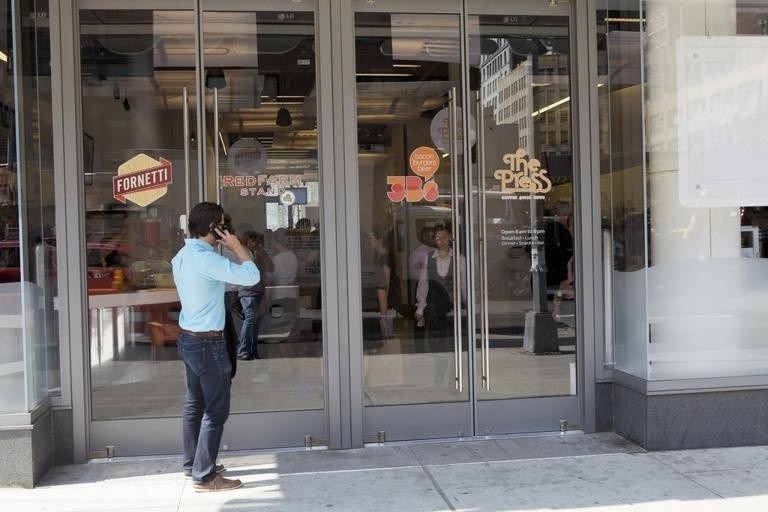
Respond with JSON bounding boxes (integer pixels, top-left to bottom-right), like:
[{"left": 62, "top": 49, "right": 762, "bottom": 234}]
[{"left": 211, "top": 225, "right": 226, "bottom": 240}]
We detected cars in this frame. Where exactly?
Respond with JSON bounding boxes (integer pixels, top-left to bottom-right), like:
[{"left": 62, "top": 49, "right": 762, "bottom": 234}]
[{"left": 125, "top": 259, "right": 174, "bottom": 291}]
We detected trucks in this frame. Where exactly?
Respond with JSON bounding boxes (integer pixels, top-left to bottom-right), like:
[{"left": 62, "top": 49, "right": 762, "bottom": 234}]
[{"left": 252, "top": 205, "right": 480, "bottom": 326}]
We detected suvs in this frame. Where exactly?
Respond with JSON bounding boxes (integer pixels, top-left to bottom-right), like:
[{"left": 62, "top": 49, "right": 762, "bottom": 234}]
[{"left": 464, "top": 190, "right": 533, "bottom": 301}]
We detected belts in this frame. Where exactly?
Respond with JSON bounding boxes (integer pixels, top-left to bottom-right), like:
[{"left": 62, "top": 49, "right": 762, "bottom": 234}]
[{"left": 181, "top": 329, "right": 225, "bottom": 337}]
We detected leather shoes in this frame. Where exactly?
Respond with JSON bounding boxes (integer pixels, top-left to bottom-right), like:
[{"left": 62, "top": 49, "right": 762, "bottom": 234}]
[
  {"left": 182, "top": 461, "right": 228, "bottom": 479},
  {"left": 191, "top": 473, "right": 245, "bottom": 493}
]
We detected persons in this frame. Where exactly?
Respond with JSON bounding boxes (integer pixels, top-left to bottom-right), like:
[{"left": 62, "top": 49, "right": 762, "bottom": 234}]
[
  {"left": 367, "top": 227, "right": 466, "bottom": 357},
  {"left": 525, "top": 208, "right": 576, "bottom": 328},
  {"left": 170, "top": 203, "right": 259, "bottom": 490},
  {"left": 239, "top": 215, "right": 321, "bottom": 357}
]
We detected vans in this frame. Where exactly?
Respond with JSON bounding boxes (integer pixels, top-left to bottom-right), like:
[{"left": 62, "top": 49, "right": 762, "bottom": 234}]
[
  {"left": 86, "top": 241, "right": 131, "bottom": 295},
  {"left": 0, "top": 241, "right": 56, "bottom": 296}
]
